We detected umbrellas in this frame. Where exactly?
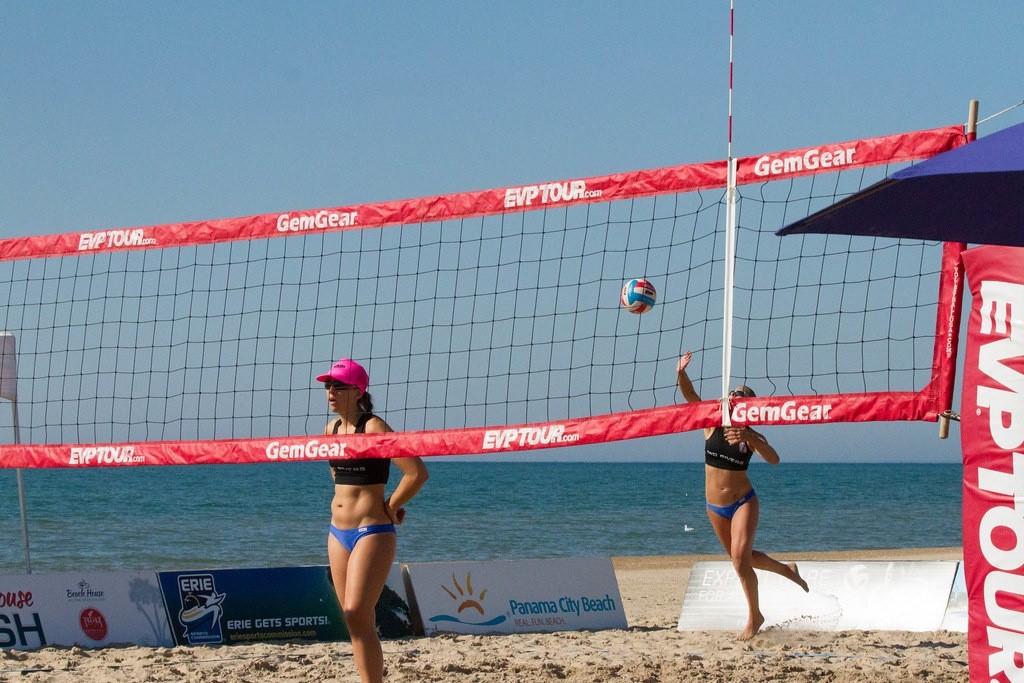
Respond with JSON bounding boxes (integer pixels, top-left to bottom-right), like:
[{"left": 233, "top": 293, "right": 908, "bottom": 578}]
[{"left": 776, "top": 122, "right": 1024, "bottom": 247}]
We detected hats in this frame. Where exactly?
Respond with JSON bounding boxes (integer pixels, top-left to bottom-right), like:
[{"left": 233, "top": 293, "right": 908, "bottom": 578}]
[{"left": 315, "top": 357, "right": 369, "bottom": 394}]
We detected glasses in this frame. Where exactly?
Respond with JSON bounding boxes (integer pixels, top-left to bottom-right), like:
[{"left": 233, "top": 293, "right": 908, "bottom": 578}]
[
  {"left": 324, "top": 378, "right": 349, "bottom": 391},
  {"left": 728, "top": 390, "right": 748, "bottom": 397}
]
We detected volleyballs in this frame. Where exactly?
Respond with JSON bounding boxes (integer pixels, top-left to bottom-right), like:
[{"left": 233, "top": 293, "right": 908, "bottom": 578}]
[{"left": 621, "top": 278, "right": 657, "bottom": 314}]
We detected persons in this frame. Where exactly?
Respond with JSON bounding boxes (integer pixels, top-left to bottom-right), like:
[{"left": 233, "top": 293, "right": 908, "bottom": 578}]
[
  {"left": 676, "top": 350, "right": 810, "bottom": 641},
  {"left": 315, "top": 357, "right": 429, "bottom": 683}
]
